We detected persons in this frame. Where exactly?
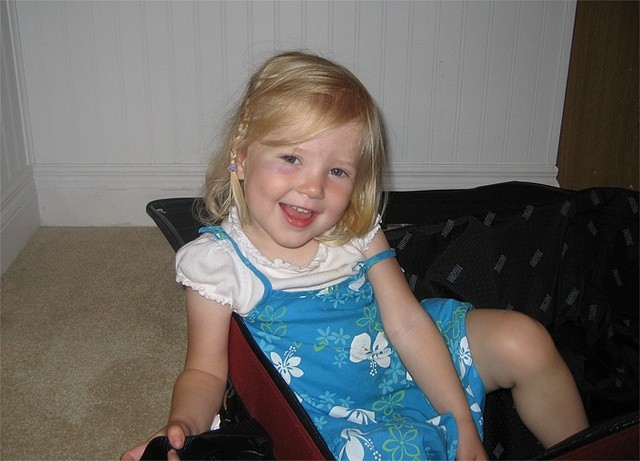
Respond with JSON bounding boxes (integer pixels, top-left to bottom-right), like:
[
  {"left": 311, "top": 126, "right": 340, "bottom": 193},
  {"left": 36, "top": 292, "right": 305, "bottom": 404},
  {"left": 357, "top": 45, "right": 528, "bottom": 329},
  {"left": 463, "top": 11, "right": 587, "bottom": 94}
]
[{"left": 118, "top": 50, "right": 591, "bottom": 461}]
